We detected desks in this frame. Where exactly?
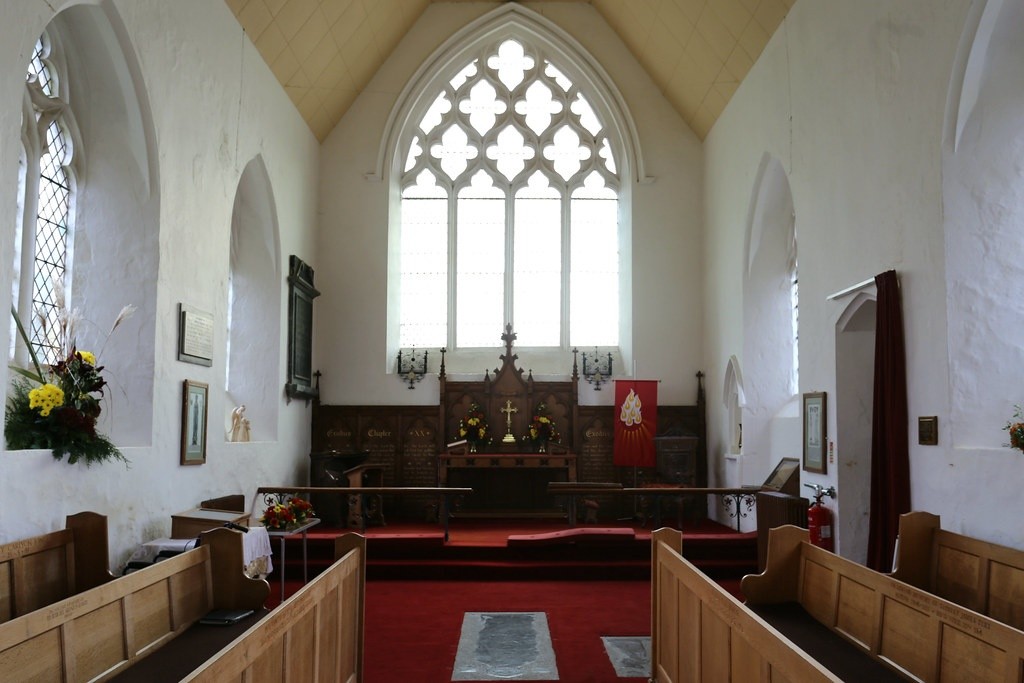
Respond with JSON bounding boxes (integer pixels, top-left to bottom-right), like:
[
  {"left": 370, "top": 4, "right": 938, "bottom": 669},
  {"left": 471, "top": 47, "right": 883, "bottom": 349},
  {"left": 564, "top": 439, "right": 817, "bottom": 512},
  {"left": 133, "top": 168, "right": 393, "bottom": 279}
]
[
  {"left": 124, "top": 523, "right": 274, "bottom": 579},
  {"left": 437, "top": 451, "right": 578, "bottom": 525},
  {"left": 639, "top": 481, "right": 688, "bottom": 531},
  {"left": 250, "top": 518, "right": 322, "bottom": 603}
]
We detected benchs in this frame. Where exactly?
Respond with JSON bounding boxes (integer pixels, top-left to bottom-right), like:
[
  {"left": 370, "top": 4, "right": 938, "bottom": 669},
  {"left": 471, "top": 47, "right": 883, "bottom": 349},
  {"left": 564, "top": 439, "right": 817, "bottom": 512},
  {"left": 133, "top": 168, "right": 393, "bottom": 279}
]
[
  {"left": 648, "top": 511, "right": 1024, "bottom": 683},
  {"left": 0, "top": 510, "right": 368, "bottom": 683}
]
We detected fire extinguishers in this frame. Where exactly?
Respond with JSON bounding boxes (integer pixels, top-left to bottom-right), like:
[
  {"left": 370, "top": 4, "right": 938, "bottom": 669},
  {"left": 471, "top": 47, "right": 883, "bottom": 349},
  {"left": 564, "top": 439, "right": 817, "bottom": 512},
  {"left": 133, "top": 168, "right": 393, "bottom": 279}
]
[{"left": 804, "top": 482, "right": 833, "bottom": 552}]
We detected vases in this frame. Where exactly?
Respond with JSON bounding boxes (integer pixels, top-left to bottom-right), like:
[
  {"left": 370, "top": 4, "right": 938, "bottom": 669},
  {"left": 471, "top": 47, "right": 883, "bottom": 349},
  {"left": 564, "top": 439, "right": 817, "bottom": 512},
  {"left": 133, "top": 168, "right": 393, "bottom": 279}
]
[{"left": 536, "top": 440, "right": 547, "bottom": 453}]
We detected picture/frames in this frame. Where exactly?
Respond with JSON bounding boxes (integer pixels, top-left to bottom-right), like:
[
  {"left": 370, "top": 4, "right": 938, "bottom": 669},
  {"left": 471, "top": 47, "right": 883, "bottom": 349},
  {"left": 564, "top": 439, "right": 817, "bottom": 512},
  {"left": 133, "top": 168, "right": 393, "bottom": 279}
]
[
  {"left": 469, "top": 437, "right": 477, "bottom": 454},
  {"left": 180, "top": 379, "right": 209, "bottom": 466},
  {"left": 802, "top": 392, "right": 827, "bottom": 475},
  {"left": 918, "top": 415, "right": 939, "bottom": 445}
]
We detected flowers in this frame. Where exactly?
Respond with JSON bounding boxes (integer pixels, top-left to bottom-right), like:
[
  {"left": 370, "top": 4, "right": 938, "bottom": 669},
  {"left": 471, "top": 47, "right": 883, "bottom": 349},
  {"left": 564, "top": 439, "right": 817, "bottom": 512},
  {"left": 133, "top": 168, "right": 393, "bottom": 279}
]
[
  {"left": 1001, "top": 405, "right": 1024, "bottom": 454},
  {"left": 523, "top": 403, "right": 559, "bottom": 442},
  {"left": 258, "top": 496, "right": 315, "bottom": 532},
  {"left": 459, "top": 402, "right": 488, "bottom": 440},
  {"left": 2, "top": 277, "right": 138, "bottom": 471}
]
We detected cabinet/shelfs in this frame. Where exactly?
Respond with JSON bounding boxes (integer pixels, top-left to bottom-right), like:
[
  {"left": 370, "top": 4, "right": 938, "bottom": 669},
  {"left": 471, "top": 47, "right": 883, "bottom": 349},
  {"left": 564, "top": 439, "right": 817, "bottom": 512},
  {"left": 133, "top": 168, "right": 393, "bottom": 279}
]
[{"left": 655, "top": 436, "right": 699, "bottom": 519}]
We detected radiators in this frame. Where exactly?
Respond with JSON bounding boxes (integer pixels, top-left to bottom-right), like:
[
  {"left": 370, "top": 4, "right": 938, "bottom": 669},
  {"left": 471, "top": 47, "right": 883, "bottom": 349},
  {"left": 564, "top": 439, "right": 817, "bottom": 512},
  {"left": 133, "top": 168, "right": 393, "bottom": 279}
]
[{"left": 755, "top": 491, "right": 809, "bottom": 574}]
